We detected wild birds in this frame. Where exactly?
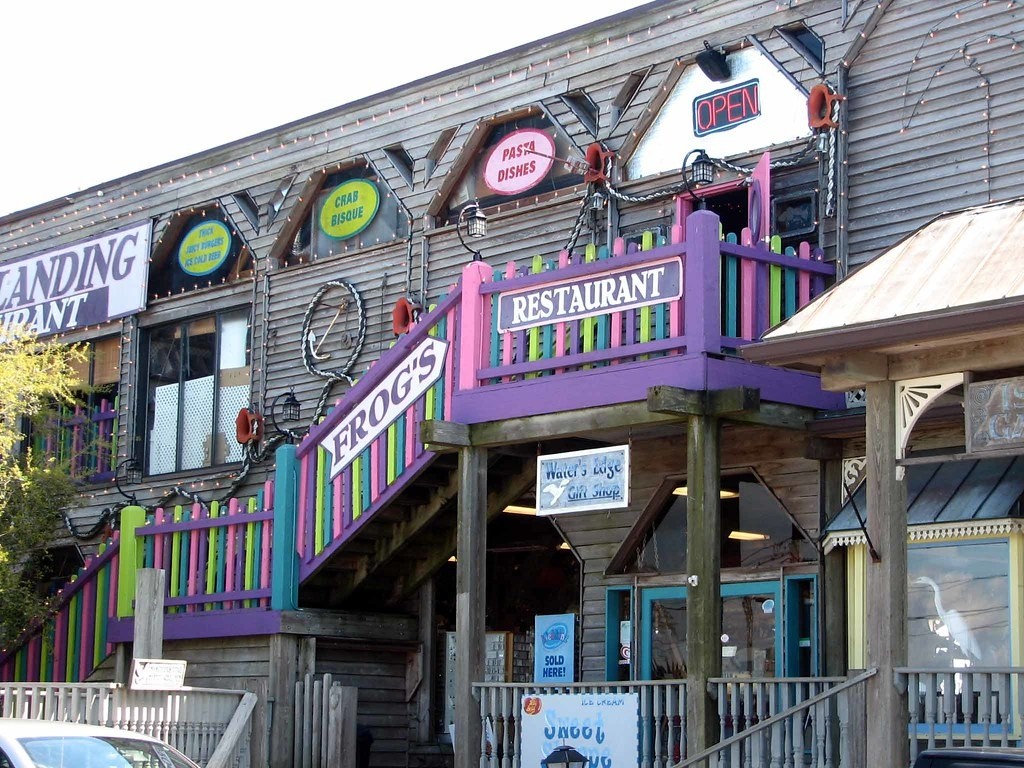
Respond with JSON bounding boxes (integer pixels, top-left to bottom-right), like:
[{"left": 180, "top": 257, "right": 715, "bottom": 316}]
[{"left": 910, "top": 575, "right": 982, "bottom": 667}]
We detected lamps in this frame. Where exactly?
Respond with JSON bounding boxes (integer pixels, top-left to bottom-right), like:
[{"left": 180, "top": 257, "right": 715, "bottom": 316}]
[
  {"left": 541, "top": 744, "right": 590, "bottom": 768},
  {"left": 114, "top": 456, "right": 144, "bottom": 506},
  {"left": 681, "top": 148, "right": 714, "bottom": 210},
  {"left": 457, "top": 196, "right": 487, "bottom": 262},
  {"left": 271, "top": 386, "right": 302, "bottom": 444}
]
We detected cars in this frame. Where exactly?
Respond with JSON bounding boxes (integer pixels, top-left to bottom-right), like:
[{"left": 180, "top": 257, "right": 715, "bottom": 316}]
[{"left": 0, "top": 717, "right": 204, "bottom": 768}]
